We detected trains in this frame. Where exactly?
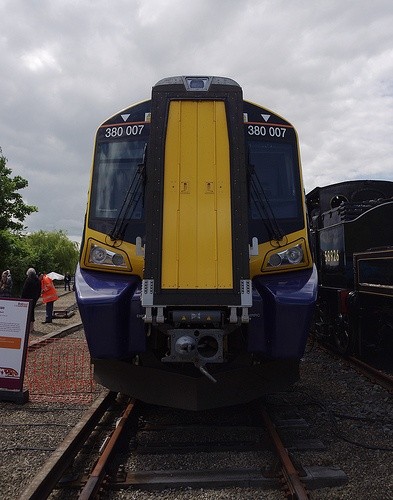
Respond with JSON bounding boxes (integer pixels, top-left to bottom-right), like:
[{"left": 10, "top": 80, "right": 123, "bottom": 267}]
[
  {"left": 303, "top": 178, "right": 393, "bottom": 380},
  {"left": 74, "top": 75, "right": 319, "bottom": 412}
]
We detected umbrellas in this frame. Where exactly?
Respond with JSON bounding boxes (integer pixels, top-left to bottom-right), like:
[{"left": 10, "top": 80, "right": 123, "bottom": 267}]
[{"left": 45, "top": 272, "right": 64, "bottom": 280}]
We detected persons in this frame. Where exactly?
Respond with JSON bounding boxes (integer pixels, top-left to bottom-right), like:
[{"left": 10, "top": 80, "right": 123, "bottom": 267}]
[
  {"left": 0, "top": 268, "right": 76, "bottom": 299},
  {"left": 21, "top": 268, "right": 41, "bottom": 331},
  {"left": 38, "top": 272, "right": 58, "bottom": 324}
]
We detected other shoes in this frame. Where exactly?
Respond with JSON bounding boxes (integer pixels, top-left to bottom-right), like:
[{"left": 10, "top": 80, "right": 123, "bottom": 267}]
[{"left": 42, "top": 321, "right": 49, "bottom": 324}]
